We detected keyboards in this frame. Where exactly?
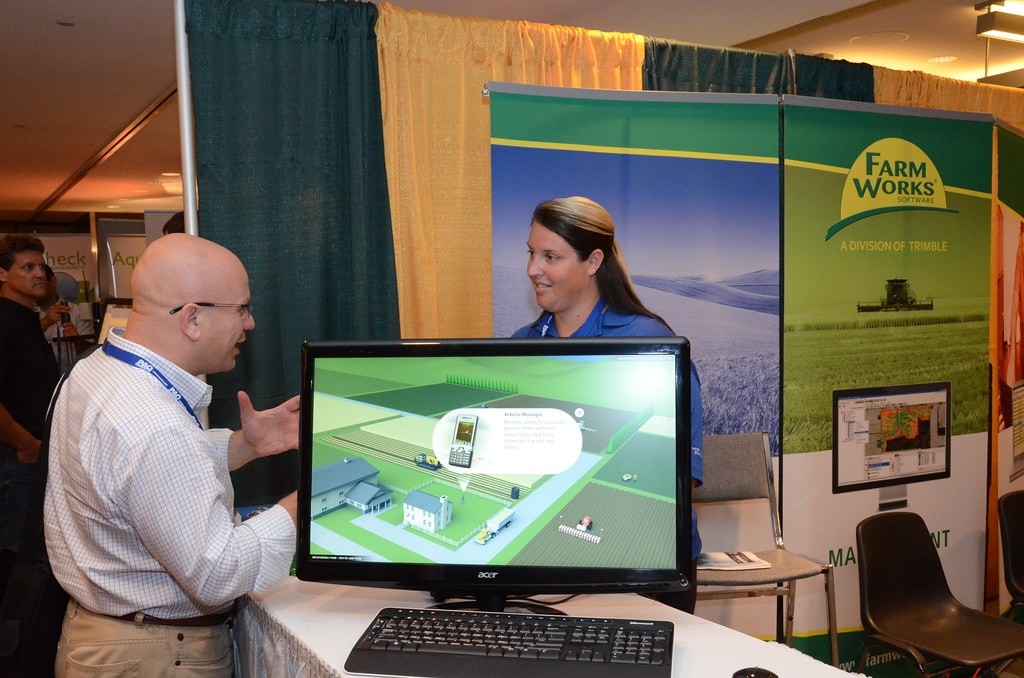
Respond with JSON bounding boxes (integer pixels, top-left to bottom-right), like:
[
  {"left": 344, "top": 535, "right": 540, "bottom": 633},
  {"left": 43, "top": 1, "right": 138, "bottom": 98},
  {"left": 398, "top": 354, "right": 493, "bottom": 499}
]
[{"left": 344, "top": 608, "right": 674, "bottom": 678}]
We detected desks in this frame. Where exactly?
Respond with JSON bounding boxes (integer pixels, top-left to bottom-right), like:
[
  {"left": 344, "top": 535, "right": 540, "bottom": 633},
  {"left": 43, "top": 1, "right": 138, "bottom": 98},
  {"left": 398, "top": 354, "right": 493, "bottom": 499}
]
[{"left": 235, "top": 574, "right": 864, "bottom": 677}]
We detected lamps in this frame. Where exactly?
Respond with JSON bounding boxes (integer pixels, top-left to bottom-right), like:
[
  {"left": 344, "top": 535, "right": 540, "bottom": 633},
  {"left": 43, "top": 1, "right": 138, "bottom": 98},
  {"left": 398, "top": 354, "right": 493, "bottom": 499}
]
[{"left": 974, "top": 1, "right": 1024, "bottom": 43}]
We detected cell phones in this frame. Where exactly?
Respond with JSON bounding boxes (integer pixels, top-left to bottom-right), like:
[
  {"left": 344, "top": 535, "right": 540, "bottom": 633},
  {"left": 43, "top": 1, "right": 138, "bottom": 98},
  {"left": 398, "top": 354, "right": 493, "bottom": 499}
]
[{"left": 449, "top": 415, "right": 479, "bottom": 468}]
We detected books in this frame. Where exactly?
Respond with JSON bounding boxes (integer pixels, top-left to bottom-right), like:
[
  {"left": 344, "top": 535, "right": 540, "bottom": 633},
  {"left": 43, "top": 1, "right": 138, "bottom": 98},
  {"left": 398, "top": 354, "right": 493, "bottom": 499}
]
[{"left": 697, "top": 551, "right": 772, "bottom": 570}]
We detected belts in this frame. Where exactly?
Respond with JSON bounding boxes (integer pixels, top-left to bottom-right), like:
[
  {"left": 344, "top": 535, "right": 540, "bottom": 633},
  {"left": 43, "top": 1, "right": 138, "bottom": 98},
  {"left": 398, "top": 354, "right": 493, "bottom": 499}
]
[{"left": 102, "top": 609, "right": 239, "bottom": 627}]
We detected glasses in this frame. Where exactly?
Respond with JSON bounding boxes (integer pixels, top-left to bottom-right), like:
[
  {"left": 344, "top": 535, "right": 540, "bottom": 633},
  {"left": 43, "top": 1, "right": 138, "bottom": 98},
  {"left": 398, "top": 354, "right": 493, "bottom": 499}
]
[{"left": 170, "top": 301, "right": 253, "bottom": 319}]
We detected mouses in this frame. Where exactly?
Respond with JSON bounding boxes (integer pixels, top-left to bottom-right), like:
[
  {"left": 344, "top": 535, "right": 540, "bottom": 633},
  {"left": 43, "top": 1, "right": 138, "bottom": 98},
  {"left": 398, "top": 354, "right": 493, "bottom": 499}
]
[{"left": 732, "top": 667, "right": 779, "bottom": 678}]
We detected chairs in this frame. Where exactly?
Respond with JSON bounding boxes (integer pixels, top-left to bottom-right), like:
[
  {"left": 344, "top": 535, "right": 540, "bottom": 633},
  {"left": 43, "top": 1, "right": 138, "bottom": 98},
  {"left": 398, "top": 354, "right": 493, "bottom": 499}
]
[
  {"left": 857, "top": 511, "right": 1024, "bottom": 678},
  {"left": 998, "top": 490, "right": 1024, "bottom": 623},
  {"left": 683, "top": 432, "right": 842, "bottom": 668}
]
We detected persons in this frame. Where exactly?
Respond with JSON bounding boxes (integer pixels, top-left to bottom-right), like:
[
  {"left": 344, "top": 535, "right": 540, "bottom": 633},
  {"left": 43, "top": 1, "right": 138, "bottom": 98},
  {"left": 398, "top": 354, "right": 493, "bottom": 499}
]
[
  {"left": 44, "top": 233, "right": 300, "bottom": 678},
  {"left": 163, "top": 209, "right": 199, "bottom": 236},
  {"left": 0, "top": 236, "right": 71, "bottom": 678},
  {"left": 508, "top": 196, "right": 703, "bottom": 615},
  {"left": 32, "top": 264, "right": 96, "bottom": 374}
]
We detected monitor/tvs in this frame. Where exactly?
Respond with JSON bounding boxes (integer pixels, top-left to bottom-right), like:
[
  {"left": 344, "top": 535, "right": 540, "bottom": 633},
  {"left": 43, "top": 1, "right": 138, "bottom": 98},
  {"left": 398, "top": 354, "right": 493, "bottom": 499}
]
[
  {"left": 298, "top": 336, "right": 692, "bottom": 616},
  {"left": 832, "top": 381, "right": 952, "bottom": 505},
  {"left": 96, "top": 298, "right": 132, "bottom": 345}
]
